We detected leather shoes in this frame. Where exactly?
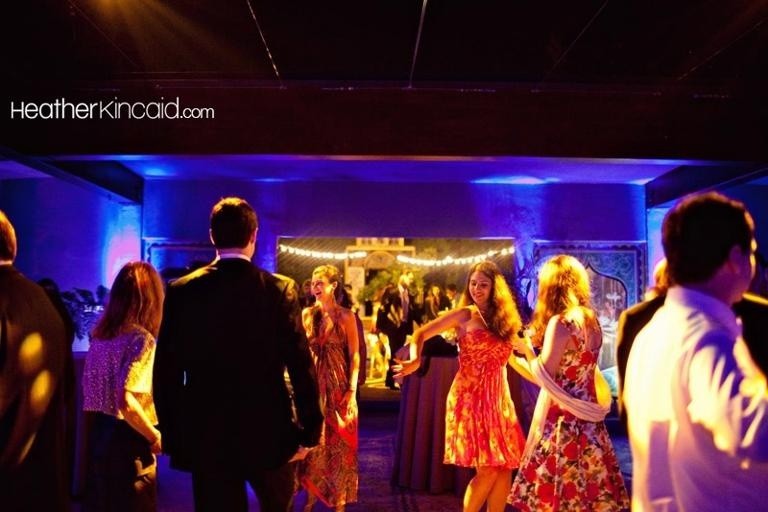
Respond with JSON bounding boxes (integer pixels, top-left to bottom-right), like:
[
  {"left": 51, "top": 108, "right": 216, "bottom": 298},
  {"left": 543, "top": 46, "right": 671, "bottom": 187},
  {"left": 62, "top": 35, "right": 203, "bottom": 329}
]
[{"left": 385, "top": 384, "right": 399, "bottom": 390}]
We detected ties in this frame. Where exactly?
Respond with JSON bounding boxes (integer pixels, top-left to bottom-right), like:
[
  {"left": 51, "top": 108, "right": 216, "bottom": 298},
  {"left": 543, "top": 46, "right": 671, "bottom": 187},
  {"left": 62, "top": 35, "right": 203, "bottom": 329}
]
[{"left": 403, "top": 295, "right": 408, "bottom": 319}]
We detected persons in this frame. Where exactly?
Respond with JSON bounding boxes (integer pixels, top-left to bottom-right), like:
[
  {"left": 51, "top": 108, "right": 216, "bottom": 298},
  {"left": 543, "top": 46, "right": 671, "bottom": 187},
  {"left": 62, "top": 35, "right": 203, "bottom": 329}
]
[{"left": 1, "top": 192, "right": 767, "bottom": 510}]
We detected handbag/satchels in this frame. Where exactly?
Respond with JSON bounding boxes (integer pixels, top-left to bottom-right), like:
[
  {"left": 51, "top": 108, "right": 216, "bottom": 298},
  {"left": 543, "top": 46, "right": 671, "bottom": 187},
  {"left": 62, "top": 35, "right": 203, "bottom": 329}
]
[
  {"left": 377, "top": 310, "right": 395, "bottom": 334},
  {"left": 423, "top": 335, "right": 458, "bottom": 357}
]
